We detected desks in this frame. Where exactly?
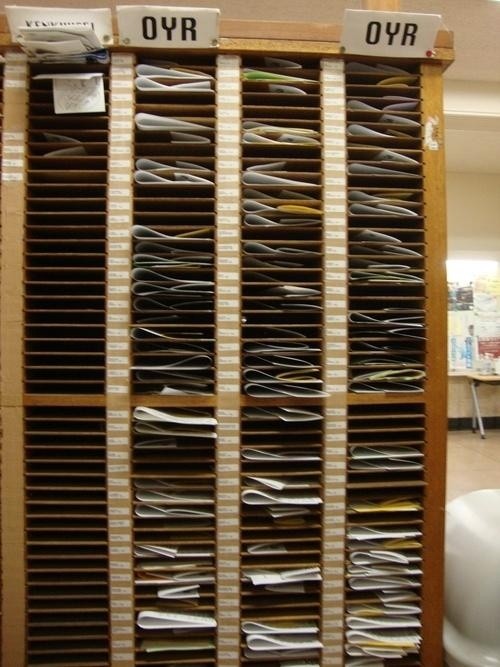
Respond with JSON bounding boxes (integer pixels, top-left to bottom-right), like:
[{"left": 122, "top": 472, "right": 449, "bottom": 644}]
[{"left": 448, "top": 372, "right": 500, "bottom": 438}]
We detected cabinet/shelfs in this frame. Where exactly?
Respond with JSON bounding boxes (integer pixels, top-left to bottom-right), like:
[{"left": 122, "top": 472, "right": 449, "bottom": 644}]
[{"left": 1, "top": 16, "right": 453, "bottom": 667}]
